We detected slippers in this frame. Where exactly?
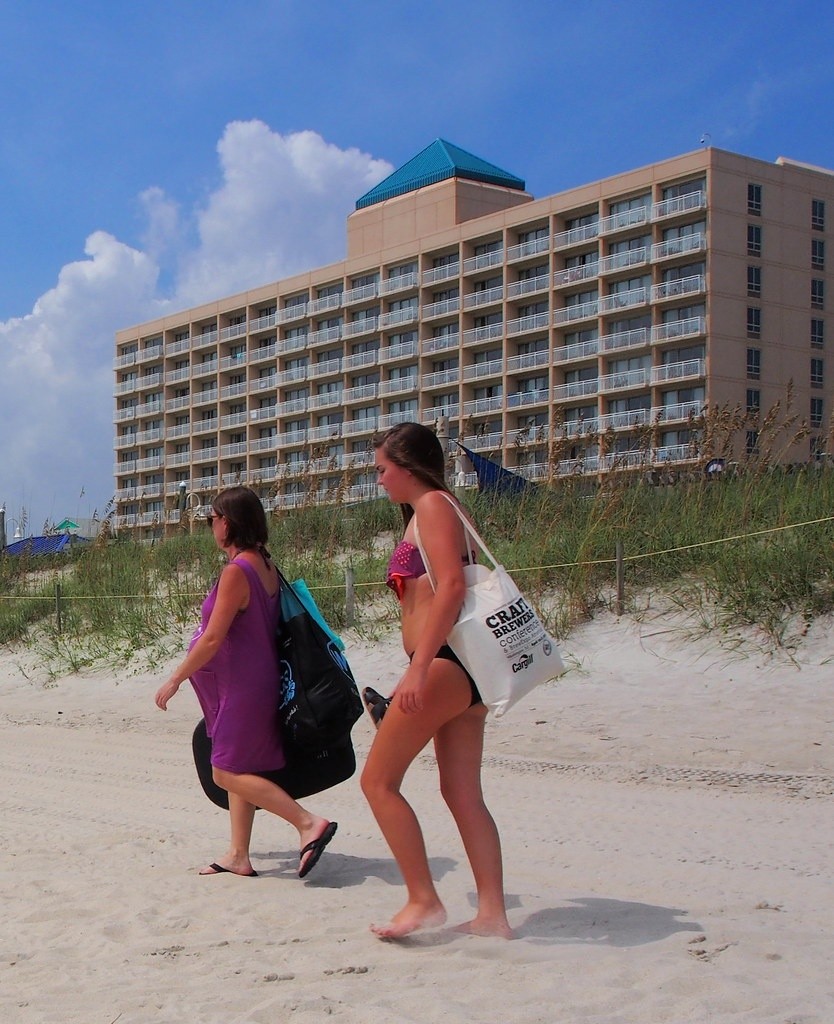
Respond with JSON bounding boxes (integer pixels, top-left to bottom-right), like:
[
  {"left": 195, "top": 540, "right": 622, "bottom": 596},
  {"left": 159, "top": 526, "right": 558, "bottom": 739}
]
[
  {"left": 361, "top": 686, "right": 395, "bottom": 729},
  {"left": 199, "top": 863, "right": 258, "bottom": 877},
  {"left": 298, "top": 822, "right": 338, "bottom": 879}
]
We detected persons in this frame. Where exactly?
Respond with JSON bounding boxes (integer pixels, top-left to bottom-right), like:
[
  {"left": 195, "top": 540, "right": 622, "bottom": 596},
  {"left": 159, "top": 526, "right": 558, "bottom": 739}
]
[
  {"left": 358, "top": 421, "right": 516, "bottom": 942},
  {"left": 153, "top": 486, "right": 341, "bottom": 880}
]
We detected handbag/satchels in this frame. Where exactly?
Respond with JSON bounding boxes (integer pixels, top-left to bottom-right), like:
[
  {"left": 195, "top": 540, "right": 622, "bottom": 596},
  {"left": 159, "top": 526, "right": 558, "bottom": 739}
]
[
  {"left": 413, "top": 493, "right": 567, "bottom": 718},
  {"left": 192, "top": 716, "right": 357, "bottom": 810},
  {"left": 274, "top": 564, "right": 364, "bottom": 749}
]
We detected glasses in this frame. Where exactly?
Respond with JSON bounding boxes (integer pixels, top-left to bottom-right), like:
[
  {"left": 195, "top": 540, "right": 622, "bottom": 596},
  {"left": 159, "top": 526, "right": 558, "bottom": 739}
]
[{"left": 207, "top": 515, "right": 226, "bottom": 527}]
